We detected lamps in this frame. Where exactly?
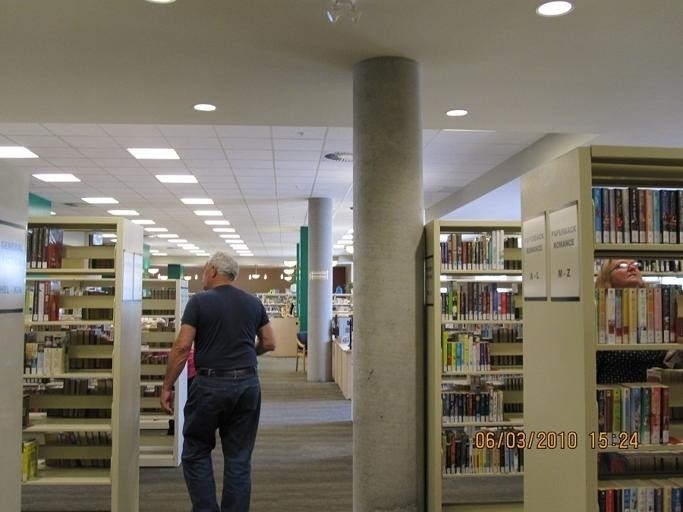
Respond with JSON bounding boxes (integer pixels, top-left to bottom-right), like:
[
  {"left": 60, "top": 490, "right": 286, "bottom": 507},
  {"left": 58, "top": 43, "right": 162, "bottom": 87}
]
[{"left": 323, "top": 2, "right": 366, "bottom": 26}]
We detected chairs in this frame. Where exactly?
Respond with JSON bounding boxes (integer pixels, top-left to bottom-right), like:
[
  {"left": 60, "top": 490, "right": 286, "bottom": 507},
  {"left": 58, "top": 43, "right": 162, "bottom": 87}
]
[{"left": 294, "top": 331, "right": 307, "bottom": 372}]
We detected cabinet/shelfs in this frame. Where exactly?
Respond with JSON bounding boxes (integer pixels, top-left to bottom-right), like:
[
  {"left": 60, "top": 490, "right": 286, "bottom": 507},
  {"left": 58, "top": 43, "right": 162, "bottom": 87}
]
[
  {"left": 0, "top": 159, "right": 32, "bottom": 512},
  {"left": 423, "top": 216, "right": 522, "bottom": 512},
  {"left": 332, "top": 293, "right": 352, "bottom": 317},
  {"left": 22, "top": 276, "right": 192, "bottom": 474},
  {"left": 517, "top": 140, "right": 682, "bottom": 510},
  {"left": 18, "top": 212, "right": 145, "bottom": 509},
  {"left": 257, "top": 291, "right": 296, "bottom": 318}
]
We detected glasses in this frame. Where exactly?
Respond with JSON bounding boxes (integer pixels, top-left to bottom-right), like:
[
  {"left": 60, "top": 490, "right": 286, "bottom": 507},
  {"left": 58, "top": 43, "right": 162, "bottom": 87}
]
[{"left": 609, "top": 261, "right": 641, "bottom": 273}]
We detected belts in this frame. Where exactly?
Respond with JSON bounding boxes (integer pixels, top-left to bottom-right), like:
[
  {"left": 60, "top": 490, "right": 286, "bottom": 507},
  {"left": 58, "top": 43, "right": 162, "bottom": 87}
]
[{"left": 197, "top": 366, "right": 255, "bottom": 376}]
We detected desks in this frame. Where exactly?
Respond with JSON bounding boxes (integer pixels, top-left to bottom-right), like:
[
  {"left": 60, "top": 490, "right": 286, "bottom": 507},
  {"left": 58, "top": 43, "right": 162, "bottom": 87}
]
[{"left": 330, "top": 339, "right": 352, "bottom": 399}]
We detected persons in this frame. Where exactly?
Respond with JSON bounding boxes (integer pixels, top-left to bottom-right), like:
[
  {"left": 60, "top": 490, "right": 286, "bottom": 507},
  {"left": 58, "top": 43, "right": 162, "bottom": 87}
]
[{"left": 160, "top": 252, "right": 275, "bottom": 512}]
